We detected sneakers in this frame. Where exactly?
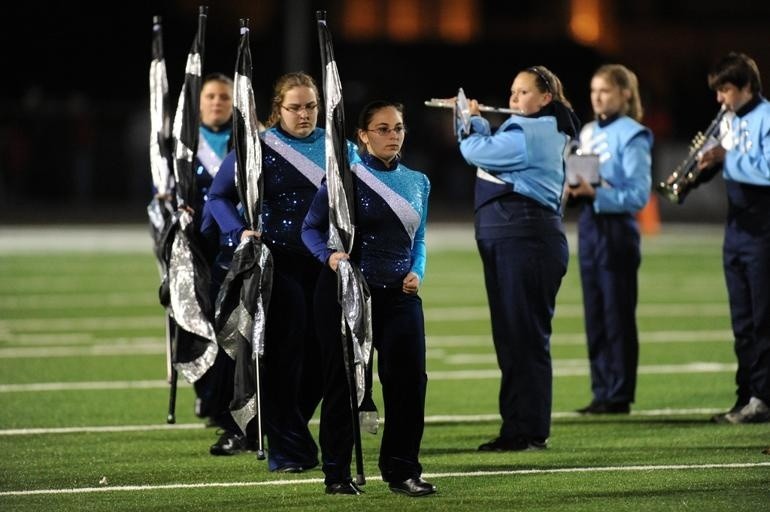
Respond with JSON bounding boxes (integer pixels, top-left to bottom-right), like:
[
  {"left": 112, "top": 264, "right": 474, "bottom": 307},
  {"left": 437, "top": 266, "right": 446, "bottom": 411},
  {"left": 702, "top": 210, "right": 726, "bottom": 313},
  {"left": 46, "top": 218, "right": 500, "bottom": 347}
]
[
  {"left": 579, "top": 397, "right": 631, "bottom": 414},
  {"left": 725, "top": 397, "right": 770, "bottom": 423},
  {"left": 210, "top": 428, "right": 244, "bottom": 456},
  {"left": 476, "top": 436, "right": 532, "bottom": 451},
  {"left": 711, "top": 400, "right": 750, "bottom": 424},
  {"left": 194, "top": 397, "right": 208, "bottom": 418},
  {"left": 529, "top": 437, "right": 548, "bottom": 450}
]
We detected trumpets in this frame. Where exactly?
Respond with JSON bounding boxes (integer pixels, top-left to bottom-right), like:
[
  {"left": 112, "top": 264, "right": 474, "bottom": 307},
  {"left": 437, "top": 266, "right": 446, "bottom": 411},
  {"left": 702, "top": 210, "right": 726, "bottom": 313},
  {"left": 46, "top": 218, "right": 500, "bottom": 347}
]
[{"left": 657, "top": 104, "right": 729, "bottom": 204}]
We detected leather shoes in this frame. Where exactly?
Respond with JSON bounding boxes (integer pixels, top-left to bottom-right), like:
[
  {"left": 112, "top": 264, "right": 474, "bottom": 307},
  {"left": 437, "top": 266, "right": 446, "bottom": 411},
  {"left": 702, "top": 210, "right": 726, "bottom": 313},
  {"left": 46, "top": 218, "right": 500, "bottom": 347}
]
[
  {"left": 388, "top": 475, "right": 438, "bottom": 497},
  {"left": 324, "top": 480, "right": 367, "bottom": 495},
  {"left": 278, "top": 461, "right": 302, "bottom": 473}
]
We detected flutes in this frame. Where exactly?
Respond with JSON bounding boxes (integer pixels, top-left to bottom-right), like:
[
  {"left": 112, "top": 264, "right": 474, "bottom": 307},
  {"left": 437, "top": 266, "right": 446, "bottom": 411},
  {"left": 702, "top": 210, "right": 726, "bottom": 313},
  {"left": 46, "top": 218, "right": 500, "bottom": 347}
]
[{"left": 424, "top": 100, "right": 525, "bottom": 114}]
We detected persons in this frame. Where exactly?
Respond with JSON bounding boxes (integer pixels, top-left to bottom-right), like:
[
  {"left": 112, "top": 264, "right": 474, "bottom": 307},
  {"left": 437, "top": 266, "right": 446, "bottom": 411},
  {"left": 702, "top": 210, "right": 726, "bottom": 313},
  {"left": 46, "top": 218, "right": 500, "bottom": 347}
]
[
  {"left": 205, "top": 70, "right": 361, "bottom": 473},
  {"left": 152, "top": 76, "right": 235, "bottom": 422},
  {"left": 300, "top": 97, "right": 438, "bottom": 497},
  {"left": 562, "top": 64, "right": 654, "bottom": 414},
  {"left": 698, "top": 52, "right": 770, "bottom": 425},
  {"left": 457, "top": 65, "right": 569, "bottom": 451}
]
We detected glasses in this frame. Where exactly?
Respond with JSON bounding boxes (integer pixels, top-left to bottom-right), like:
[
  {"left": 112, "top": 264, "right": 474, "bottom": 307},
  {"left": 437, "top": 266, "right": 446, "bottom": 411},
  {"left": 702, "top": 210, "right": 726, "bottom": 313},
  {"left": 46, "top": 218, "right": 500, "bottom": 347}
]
[
  {"left": 365, "top": 125, "right": 406, "bottom": 136},
  {"left": 278, "top": 102, "right": 320, "bottom": 112}
]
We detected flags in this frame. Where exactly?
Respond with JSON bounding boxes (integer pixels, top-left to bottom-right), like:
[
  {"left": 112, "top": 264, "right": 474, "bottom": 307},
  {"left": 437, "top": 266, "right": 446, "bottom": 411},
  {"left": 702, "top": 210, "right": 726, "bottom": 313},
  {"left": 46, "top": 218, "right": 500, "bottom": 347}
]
[
  {"left": 142, "top": 16, "right": 173, "bottom": 312},
  {"left": 212, "top": 18, "right": 275, "bottom": 433},
  {"left": 317, "top": 11, "right": 377, "bottom": 433},
  {"left": 169, "top": 7, "right": 219, "bottom": 387}
]
